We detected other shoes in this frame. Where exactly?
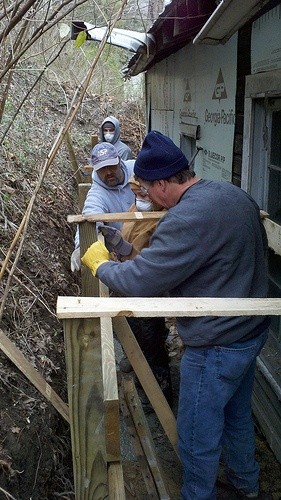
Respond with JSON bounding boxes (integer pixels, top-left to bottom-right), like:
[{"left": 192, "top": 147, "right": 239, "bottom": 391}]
[
  {"left": 117, "top": 352, "right": 130, "bottom": 372},
  {"left": 214, "top": 462, "right": 248, "bottom": 500},
  {"left": 140, "top": 398, "right": 152, "bottom": 412}
]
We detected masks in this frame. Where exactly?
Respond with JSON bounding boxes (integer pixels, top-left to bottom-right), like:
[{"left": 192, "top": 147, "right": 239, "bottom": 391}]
[
  {"left": 104, "top": 134, "right": 114, "bottom": 142},
  {"left": 135, "top": 198, "right": 153, "bottom": 211}
]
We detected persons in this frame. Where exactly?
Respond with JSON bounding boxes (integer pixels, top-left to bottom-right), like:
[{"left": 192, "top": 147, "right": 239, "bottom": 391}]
[
  {"left": 70, "top": 116, "right": 174, "bottom": 414},
  {"left": 80, "top": 129, "right": 272, "bottom": 500}
]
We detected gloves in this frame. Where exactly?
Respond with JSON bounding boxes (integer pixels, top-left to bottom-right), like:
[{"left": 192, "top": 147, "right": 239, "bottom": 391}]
[
  {"left": 70, "top": 247, "right": 82, "bottom": 273},
  {"left": 98, "top": 224, "right": 133, "bottom": 255},
  {"left": 80, "top": 239, "right": 110, "bottom": 277}
]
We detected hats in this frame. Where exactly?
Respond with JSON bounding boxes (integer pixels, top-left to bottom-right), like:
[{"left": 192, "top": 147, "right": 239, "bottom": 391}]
[
  {"left": 90, "top": 141, "right": 119, "bottom": 172},
  {"left": 102, "top": 122, "right": 115, "bottom": 130},
  {"left": 134, "top": 130, "right": 189, "bottom": 179}
]
